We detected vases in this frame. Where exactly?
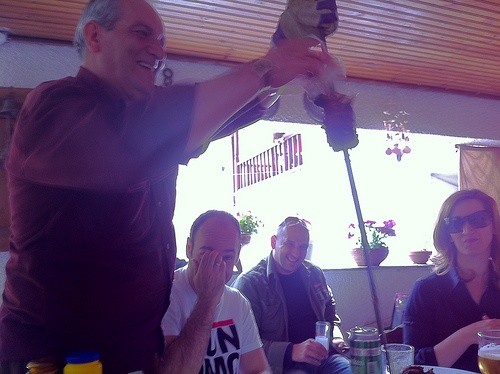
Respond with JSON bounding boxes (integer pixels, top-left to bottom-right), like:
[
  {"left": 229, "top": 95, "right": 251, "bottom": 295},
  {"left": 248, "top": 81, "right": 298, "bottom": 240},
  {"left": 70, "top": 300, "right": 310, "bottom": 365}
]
[
  {"left": 241, "top": 235, "right": 252, "bottom": 243},
  {"left": 352, "top": 247, "right": 389, "bottom": 266},
  {"left": 408, "top": 249, "right": 432, "bottom": 263}
]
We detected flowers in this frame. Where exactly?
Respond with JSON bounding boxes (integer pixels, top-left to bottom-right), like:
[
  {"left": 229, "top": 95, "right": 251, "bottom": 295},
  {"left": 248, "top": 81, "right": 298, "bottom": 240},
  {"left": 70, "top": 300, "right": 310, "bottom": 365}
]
[
  {"left": 236, "top": 210, "right": 264, "bottom": 234},
  {"left": 347, "top": 221, "right": 397, "bottom": 248}
]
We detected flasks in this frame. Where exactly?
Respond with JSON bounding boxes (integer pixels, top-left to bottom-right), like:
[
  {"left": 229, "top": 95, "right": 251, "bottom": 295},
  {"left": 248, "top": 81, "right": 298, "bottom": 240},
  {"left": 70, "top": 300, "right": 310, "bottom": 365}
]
[{"left": 349, "top": 328, "right": 381, "bottom": 374}]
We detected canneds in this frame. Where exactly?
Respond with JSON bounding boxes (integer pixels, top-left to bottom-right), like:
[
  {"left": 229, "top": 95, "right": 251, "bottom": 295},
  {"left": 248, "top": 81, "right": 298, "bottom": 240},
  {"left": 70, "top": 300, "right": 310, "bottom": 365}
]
[{"left": 348, "top": 325, "right": 385, "bottom": 374}]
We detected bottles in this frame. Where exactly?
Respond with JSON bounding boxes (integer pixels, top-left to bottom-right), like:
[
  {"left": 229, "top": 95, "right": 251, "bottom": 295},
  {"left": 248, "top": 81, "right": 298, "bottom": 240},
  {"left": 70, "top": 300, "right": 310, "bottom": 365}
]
[
  {"left": 63, "top": 350, "right": 103, "bottom": 374},
  {"left": 391, "top": 293, "right": 408, "bottom": 330}
]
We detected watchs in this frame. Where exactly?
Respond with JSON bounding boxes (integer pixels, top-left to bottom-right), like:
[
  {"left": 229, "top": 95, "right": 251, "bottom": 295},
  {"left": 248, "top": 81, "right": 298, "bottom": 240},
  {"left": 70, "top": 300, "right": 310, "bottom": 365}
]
[{"left": 252, "top": 58, "right": 271, "bottom": 89}]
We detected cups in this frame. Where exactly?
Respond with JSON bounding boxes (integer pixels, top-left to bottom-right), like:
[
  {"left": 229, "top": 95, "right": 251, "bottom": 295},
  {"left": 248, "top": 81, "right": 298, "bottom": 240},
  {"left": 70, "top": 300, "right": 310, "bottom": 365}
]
[
  {"left": 381, "top": 343, "right": 415, "bottom": 374},
  {"left": 477, "top": 330, "right": 500, "bottom": 374},
  {"left": 314, "top": 321, "right": 330, "bottom": 352}
]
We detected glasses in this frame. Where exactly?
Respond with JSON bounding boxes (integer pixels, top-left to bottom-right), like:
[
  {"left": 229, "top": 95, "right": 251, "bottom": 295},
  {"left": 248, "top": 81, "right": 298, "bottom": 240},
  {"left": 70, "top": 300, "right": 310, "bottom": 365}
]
[
  {"left": 279, "top": 217, "right": 312, "bottom": 228},
  {"left": 444, "top": 210, "right": 493, "bottom": 233}
]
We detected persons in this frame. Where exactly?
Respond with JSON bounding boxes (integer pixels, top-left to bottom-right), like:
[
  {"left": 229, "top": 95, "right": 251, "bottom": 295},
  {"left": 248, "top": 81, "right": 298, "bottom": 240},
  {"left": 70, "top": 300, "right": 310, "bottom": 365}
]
[
  {"left": 403, "top": 191, "right": 500, "bottom": 374},
  {"left": 0, "top": 1, "right": 354, "bottom": 374}
]
